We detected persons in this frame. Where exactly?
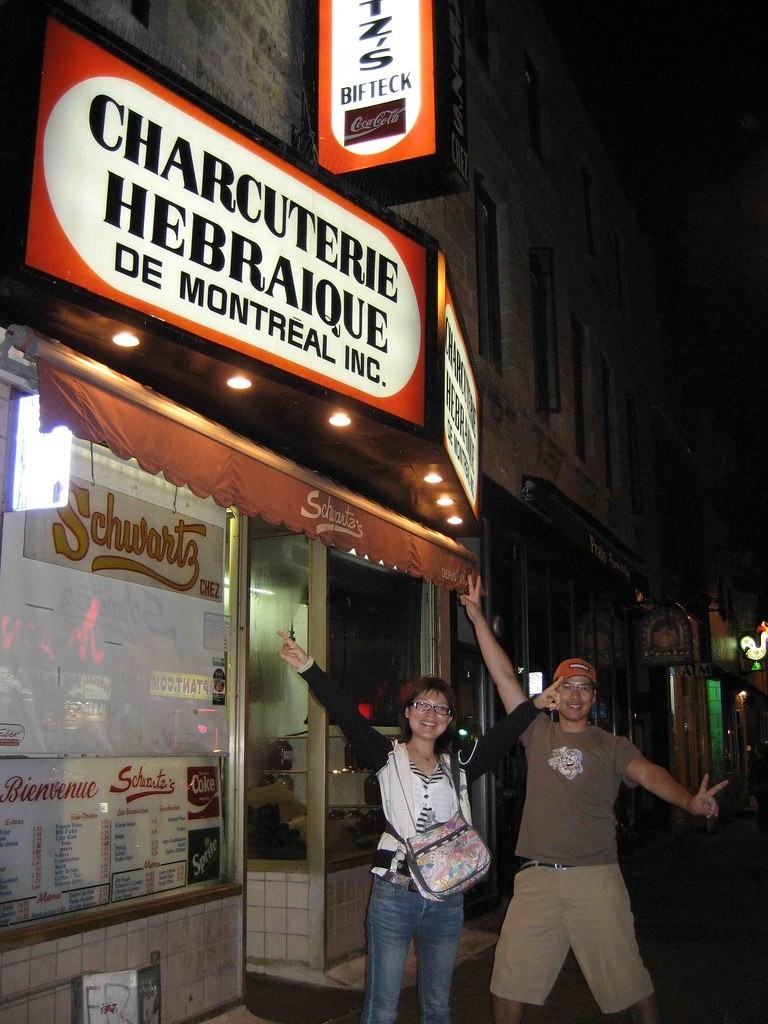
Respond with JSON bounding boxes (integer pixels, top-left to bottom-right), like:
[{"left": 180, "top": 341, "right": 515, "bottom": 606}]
[
  {"left": 277, "top": 630, "right": 564, "bottom": 1024},
  {"left": 460, "top": 575, "right": 729, "bottom": 1024},
  {"left": 248, "top": 803, "right": 307, "bottom": 860},
  {"left": 747, "top": 742, "right": 768, "bottom": 836}
]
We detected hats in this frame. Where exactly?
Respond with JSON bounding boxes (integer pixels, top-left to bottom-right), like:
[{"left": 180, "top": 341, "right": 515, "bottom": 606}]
[{"left": 553, "top": 658, "right": 597, "bottom": 683}]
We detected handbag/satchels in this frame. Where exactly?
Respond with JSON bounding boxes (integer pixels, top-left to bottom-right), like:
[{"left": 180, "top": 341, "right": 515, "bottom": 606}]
[{"left": 404, "top": 812, "right": 492, "bottom": 903}]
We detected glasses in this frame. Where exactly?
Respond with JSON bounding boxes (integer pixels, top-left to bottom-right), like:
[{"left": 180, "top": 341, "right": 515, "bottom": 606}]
[{"left": 411, "top": 702, "right": 452, "bottom": 715}]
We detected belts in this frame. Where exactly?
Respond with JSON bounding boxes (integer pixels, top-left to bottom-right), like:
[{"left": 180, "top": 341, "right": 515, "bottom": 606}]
[
  {"left": 370, "top": 867, "right": 419, "bottom": 894},
  {"left": 519, "top": 863, "right": 574, "bottom": 871}
]
